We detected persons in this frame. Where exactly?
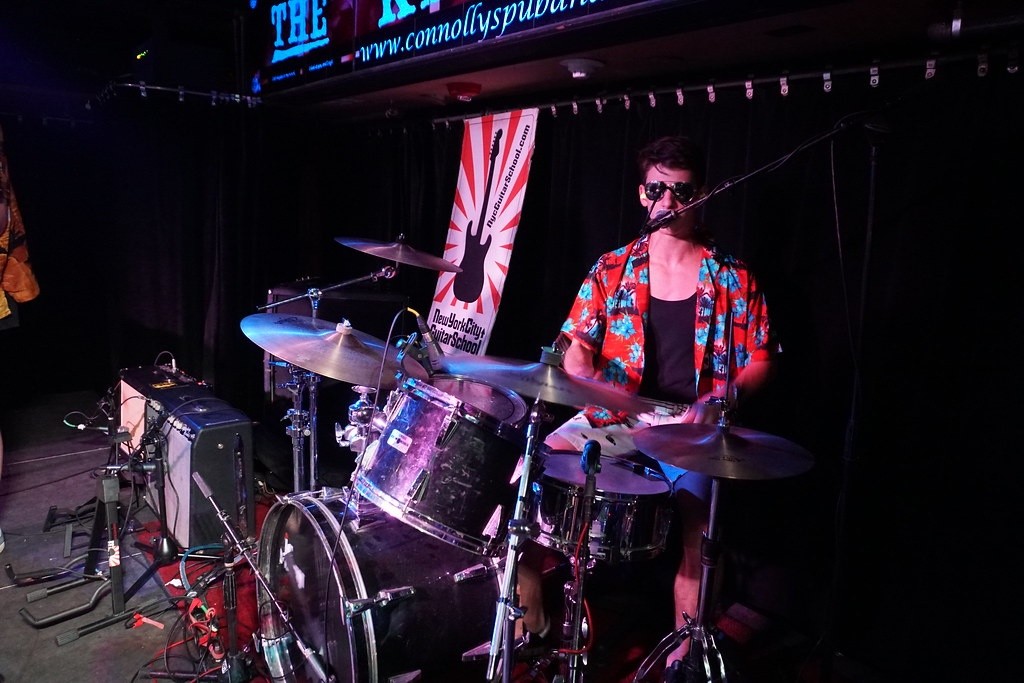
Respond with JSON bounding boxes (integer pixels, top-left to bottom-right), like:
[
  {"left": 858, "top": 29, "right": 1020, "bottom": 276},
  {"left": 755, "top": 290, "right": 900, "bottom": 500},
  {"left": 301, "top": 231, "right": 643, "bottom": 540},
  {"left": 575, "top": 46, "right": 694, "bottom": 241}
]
[{"left": 461, "top": 137, "right": 771, "bottom": 683}]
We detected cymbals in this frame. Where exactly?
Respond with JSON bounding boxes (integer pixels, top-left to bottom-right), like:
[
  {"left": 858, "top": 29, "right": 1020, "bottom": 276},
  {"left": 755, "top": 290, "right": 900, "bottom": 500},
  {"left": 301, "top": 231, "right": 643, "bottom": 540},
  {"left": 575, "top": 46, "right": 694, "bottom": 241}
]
[
  {"left": 633, "top": 422, "right": 813, "bottom": 484},
  {"left": 240, "top": 312, "right": 428, "bottom": 390},
  {"left": 339, "top": 238, "right": 464, "bottom": 274},
  {"left": 438, "top": 352, "right": 657, "bottom": 415}
]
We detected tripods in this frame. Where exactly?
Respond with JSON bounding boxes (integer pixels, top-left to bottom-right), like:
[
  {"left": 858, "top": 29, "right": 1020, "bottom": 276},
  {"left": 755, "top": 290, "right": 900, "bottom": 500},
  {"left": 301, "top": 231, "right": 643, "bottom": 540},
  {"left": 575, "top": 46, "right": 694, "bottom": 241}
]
[
  {"left": 525, "top": 497, "right": 600, "bottom": 682},
  {"left": 120, "top": 414, "right": 332, "bottom": 683},
  {"left": 630, "top": 479, "right": 730, "bottom": 683}
]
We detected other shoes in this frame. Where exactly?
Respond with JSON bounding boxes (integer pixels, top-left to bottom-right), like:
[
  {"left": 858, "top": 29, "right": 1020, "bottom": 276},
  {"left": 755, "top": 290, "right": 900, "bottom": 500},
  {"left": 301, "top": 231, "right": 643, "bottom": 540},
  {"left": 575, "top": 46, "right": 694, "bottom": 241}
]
[
  {"left": 519, "top": 613, "right": 551, "bottom": 659},
  {"left": 664, "top": 660, "right": 682, "bottom": 683}
]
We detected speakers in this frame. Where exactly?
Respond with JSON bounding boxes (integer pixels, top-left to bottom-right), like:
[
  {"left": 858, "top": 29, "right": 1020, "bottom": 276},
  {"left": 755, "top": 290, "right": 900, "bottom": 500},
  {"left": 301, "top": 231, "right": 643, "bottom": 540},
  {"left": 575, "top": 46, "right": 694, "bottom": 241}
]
[{"left": 117, "top": 367, "right": 253, "bottom": 549}]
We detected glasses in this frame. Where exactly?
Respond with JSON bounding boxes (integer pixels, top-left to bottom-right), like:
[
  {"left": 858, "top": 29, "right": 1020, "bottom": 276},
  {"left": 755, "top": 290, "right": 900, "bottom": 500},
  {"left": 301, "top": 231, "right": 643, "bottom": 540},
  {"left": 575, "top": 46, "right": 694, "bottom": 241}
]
[{"left": 645, "top": 180, "right": 697, "bottom": 203}]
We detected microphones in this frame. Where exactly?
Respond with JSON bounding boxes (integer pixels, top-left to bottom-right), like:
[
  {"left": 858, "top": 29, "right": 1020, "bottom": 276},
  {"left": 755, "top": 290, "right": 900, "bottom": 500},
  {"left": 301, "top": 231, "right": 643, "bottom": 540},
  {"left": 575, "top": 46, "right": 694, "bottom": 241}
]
[
  {"left": 416, "top": 315, "right": 447, "bottom": 369},
  {"left": 99, "top": 461, "right": 168, "bottom": 473},
  {"left": 76, "top": 424, "right": 128, "bottom": 433},
  {"left": 639, "top": 209, "right": 671, "bottom": 233},
  {"left": 579, "top": 439, "right": 601, "bottom": 522}
]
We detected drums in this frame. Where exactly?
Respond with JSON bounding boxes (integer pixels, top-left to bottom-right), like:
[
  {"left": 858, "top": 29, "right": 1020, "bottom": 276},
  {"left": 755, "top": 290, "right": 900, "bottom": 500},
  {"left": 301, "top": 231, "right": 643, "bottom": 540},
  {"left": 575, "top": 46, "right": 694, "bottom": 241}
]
[
  {"left": 426, "top": 369, "right": 528, "bottom": 423},
  {"left": 254, "top": 483, "right": 511, "bottom": 683},
  {"left": 518, "top": 444, "right": 675, "bottom": 565},
  {"left": 351, "top": 373, "right": 533, "bottom": 557}
]
